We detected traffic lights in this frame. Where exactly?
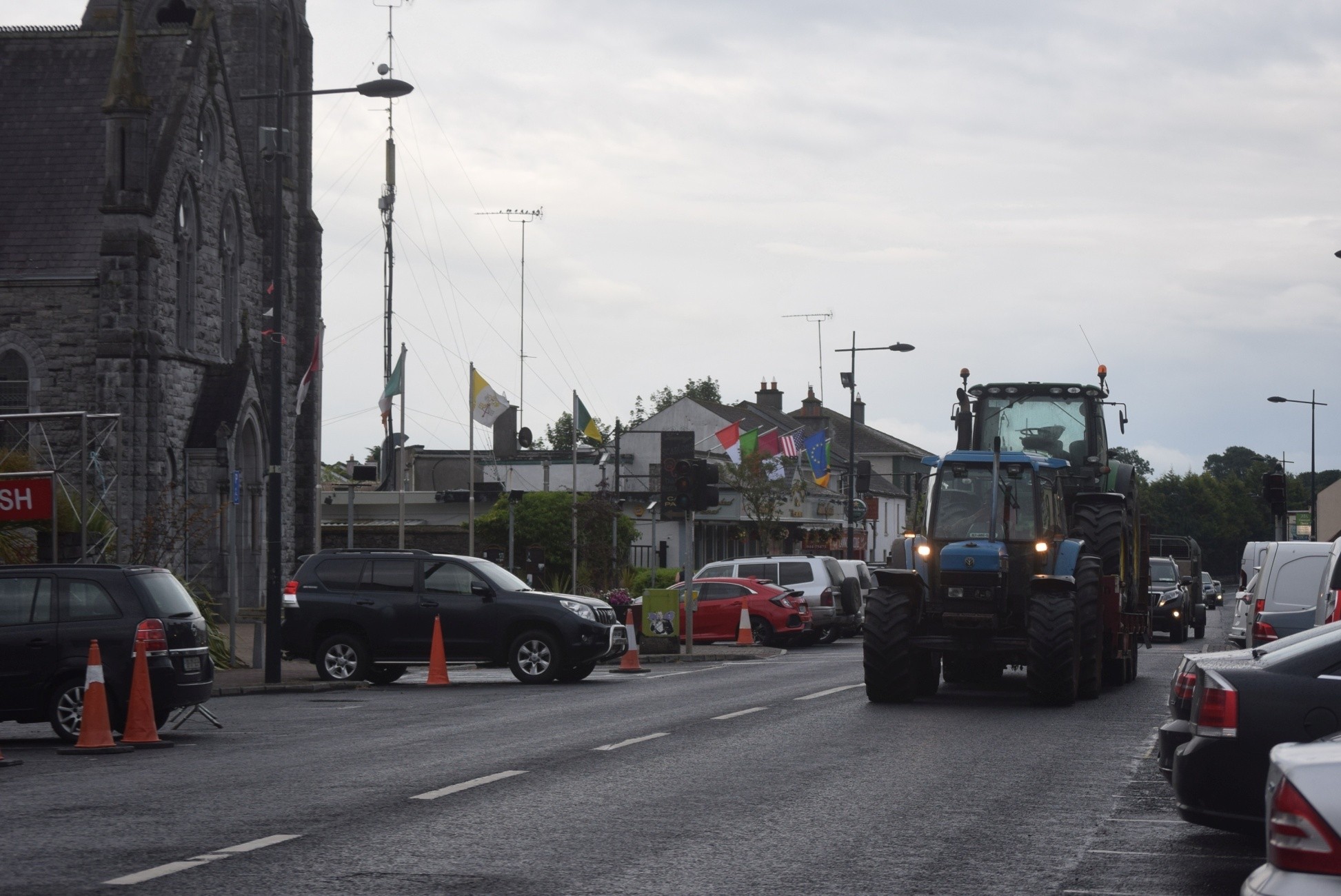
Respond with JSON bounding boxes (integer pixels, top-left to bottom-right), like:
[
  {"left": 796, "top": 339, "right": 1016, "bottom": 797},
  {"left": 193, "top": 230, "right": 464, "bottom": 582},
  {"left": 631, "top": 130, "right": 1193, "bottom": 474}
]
[
  {"left": 693, "top": 458, "right": 721, "bottom": 512},
  {"left": 674, "top": 456, "right": 696, "bottom": 513}
]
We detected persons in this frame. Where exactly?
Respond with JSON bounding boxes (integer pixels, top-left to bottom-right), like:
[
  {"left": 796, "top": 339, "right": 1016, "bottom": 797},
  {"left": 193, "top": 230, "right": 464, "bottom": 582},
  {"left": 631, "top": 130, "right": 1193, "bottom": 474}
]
[{"left": 955, "top": 489, "right": 1016, "bottom": 540}]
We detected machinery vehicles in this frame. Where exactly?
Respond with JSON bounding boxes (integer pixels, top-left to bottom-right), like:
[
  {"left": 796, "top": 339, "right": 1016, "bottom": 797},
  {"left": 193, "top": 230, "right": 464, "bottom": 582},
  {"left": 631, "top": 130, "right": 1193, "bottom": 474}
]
[
  {"left": 861, "top": 434, "right": 1139, "bottom": 713},
  {"left": 953, "top": 364, "right": 1142, "bottom": 583}
]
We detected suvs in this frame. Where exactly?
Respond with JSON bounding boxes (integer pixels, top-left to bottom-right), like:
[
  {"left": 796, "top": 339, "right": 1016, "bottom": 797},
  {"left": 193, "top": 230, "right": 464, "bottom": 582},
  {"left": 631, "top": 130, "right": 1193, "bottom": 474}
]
[
  {"left": 0, "top": 561, "right": 216, "bottom": 744},
  {"left": 280, "top": 546, "right": 629, "bottom": 685}
]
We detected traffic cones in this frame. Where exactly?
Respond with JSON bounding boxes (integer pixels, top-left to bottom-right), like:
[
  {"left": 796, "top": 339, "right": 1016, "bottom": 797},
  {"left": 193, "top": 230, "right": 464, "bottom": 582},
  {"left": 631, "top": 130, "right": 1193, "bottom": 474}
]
[
  {"left": 419, "top": 616, "right": 459, "bottom": 688},
  {"left": 727, "top": 596, "right": 764, "bottom": 648},
  {"left": 55, "top": 639, "right": 137, "bottom": 755},
  {"left": 608, "top": 608, "right": 651, "bottom": 674},
  {"left": 114, "top": 635, "right": 176, "bottom": 750}
]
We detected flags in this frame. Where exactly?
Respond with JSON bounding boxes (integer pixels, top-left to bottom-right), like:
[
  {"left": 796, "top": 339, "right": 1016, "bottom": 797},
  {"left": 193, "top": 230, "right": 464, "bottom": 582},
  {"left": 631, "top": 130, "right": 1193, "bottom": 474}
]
[
  {"left": 739, "top": 428, "right": 758, "bottom": 467},
  {"left": 782, "top": 428, "right": 804, "bottom": 465},
  {"left": 577, "top": 394, "right": 603, "bottom": 443},
  {"left": 805, "top": 440, "right": 831, "bottom": 488},
  {"left": 714, "top": 423, "right": 743, "bottom": 470},
  {"left": 805, "top": 430, "right": 829, "bottom": 479},
  {"left": 379, "top": 345, "right": 406, "bottom": 423},
  {"left": 296, "top": 321, "right": 324, "bottom": 415},
  {"left": 758, "top": 431, "right": 780, "bottom": 465},
  {"left": 472, "top": 368, "right": 511, "bottom": 428}
]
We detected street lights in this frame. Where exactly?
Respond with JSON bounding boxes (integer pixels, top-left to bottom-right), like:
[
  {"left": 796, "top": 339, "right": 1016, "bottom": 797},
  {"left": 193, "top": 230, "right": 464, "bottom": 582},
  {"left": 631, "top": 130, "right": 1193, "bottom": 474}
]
[
  {"left": 835, "top": 329, "right": 916, "bottom": 563},
  {"left": 238, "top": 76, "right": 415, "bottom": 685},
  {"left": 1266, "top": 389, "right": 1329, "bottom": 543}
]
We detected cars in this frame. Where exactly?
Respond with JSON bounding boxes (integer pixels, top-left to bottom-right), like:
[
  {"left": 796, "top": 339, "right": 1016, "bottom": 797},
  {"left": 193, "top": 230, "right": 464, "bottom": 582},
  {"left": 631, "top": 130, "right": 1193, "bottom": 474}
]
[
  {"left": 1146, "top": 532, "right": 1341, "bottom": 895},
  {"left": 630, "top": 552, "right": 895, "bottom": 649}
]
